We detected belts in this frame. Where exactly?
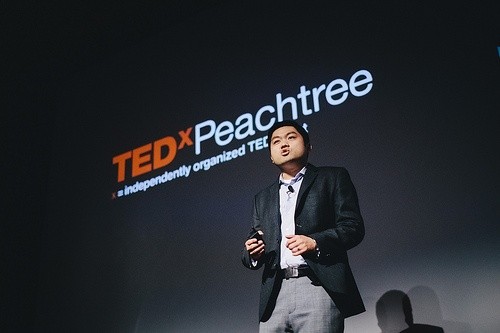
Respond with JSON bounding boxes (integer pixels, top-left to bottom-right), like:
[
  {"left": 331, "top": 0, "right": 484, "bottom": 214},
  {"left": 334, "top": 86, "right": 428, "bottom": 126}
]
[{"left": 276, "top": 266, "right": 311, "bottom": 279}]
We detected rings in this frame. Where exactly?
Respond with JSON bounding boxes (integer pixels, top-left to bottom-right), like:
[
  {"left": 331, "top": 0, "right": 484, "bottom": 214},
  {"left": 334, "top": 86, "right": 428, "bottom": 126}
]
[{"left": 296, "top": 246, "right": 299, "bottom": 251}]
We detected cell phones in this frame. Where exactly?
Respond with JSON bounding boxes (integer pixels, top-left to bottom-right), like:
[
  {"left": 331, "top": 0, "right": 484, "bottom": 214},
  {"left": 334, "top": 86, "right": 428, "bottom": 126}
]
[{"left": 249, "top": 226, "right": 266, "bottom": 246}]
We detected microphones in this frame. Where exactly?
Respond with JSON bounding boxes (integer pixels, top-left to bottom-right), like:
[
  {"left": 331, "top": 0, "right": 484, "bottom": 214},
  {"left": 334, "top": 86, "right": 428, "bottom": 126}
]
[{"left": 288, "top": 185, "right": 293, "bottom": 192}]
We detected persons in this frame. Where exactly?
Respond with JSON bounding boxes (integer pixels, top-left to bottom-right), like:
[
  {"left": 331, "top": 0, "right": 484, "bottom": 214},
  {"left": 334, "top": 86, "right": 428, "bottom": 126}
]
[{"left": 241, "top": 120, "right": 366, "bottom": 333}]
